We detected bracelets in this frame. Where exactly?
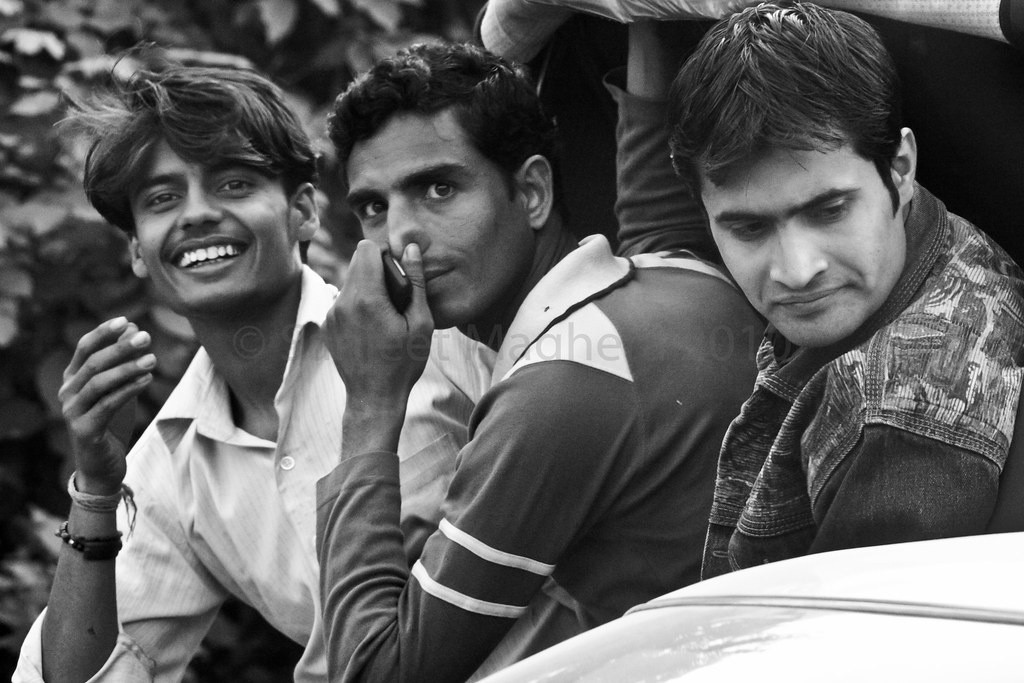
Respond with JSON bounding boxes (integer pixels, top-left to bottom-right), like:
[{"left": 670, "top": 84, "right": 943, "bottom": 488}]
[
  {"left": 68, "top": 469, "right": 134, "bottom": 512},
  {"left": 57, "top": 521, "right": 123, "bottom": 562}
]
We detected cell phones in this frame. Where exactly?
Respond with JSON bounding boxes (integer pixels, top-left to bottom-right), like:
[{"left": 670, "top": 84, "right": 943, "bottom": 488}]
[{"left": 380, "top": 253, "right": 413, "bottom": 313}]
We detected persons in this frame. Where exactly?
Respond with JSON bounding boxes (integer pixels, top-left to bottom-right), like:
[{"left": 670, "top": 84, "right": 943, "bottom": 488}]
[
  {"left": 318, "top": 12, "right": 771, "bottom": 683},
  {"left": 668, "top": 2, "right": 1024, "bottom": 580},
  {"left": 6, "top": 42, "right": 501, "bottom": 682}
]
